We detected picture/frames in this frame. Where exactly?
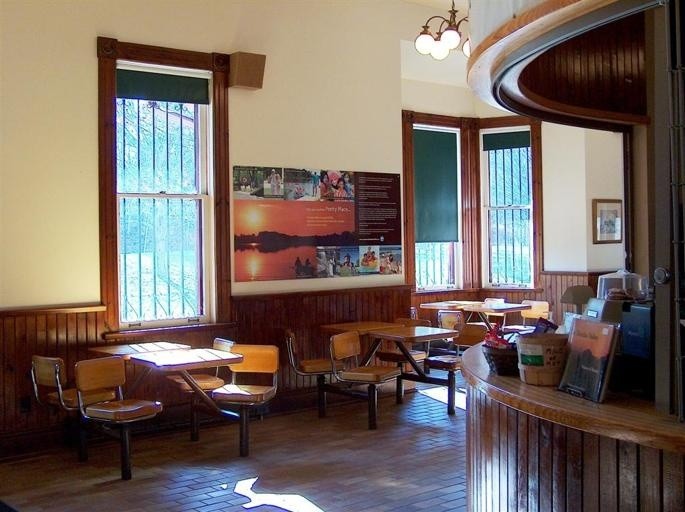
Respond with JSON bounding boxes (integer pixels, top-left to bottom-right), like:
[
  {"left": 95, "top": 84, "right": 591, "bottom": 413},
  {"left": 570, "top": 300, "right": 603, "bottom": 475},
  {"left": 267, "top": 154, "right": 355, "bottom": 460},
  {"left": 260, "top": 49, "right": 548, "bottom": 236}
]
[{"left": 591, "top": 197, "right": 624, "bottom": 245}]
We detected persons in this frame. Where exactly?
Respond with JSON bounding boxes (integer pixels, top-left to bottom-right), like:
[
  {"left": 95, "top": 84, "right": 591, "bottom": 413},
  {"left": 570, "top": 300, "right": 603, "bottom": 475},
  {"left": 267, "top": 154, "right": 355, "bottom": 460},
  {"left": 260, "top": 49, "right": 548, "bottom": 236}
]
[
  {"left": 238, "top": 169, "right": 354, "bottom": 202},
  {"left": 293, "top": 246, "right": 402, "bottom": 276}
]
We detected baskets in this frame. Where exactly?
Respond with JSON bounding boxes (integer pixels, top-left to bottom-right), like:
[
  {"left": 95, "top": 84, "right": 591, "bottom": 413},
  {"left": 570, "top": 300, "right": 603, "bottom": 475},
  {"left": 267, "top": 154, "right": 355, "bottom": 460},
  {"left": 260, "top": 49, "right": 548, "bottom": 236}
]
[{"left": 482, "top": 331, "right": 520, "bottom": 375}]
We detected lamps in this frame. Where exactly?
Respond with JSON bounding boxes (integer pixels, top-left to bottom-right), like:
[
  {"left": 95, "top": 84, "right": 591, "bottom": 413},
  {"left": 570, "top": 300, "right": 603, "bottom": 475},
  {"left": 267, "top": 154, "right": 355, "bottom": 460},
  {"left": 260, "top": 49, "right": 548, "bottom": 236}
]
[{"left": 416, "top": 1, "right": 469, "bottom": 64}]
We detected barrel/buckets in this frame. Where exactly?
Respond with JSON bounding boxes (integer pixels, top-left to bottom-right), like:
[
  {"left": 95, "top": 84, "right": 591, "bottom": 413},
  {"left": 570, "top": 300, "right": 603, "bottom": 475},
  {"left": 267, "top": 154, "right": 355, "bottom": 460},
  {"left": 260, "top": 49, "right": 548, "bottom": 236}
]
[{"left": 517, "top": 332, "right": 569, "bottom": 386}]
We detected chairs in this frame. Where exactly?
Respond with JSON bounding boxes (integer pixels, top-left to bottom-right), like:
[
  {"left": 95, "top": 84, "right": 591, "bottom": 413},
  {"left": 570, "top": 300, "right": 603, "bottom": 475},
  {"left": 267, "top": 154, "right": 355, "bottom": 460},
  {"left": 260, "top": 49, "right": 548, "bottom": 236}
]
[
  {"left": 284, "top": 292, "right": 551, "bottom": 430},
  {"left": 31, "top": 336, "right": 280, "bottom": 481}
]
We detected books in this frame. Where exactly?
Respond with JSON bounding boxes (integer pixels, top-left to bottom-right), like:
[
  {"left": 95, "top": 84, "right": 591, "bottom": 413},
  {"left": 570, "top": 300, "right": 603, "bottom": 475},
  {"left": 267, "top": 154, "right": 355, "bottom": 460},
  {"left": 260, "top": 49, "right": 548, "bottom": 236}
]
[{"left": 559, "top": 316, "right": 618, "bottom": 404}]
